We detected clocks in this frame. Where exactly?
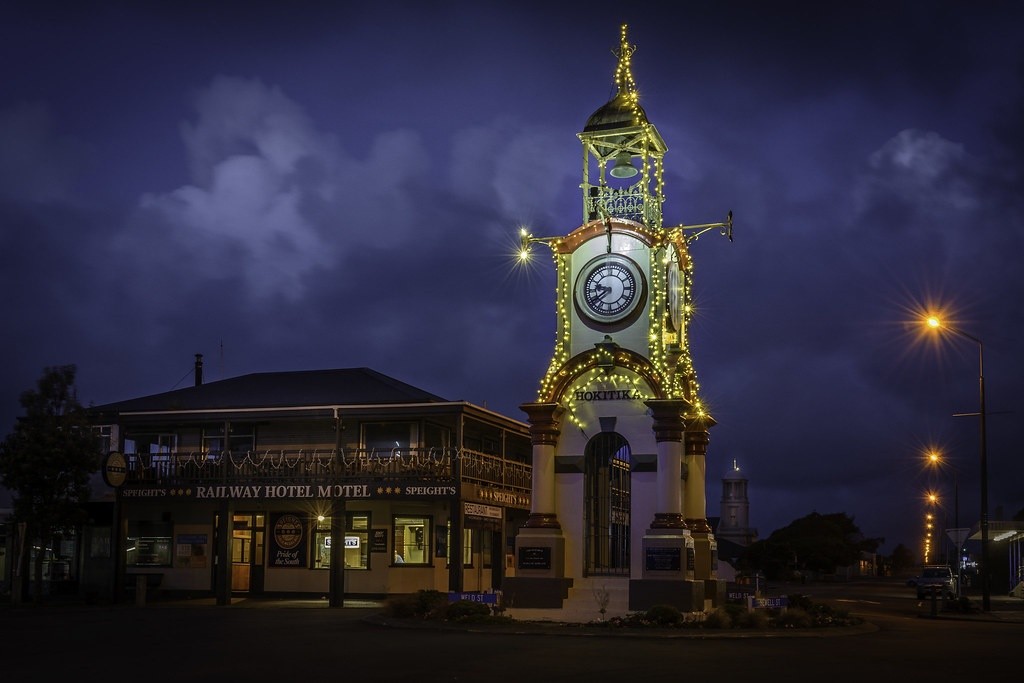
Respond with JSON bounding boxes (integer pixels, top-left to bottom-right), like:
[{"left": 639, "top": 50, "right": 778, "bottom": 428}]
[{"left": 573, "top": 253, "right": 648, "bottom": 334}]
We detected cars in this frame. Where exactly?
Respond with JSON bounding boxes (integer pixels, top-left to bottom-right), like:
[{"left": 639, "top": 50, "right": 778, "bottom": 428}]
[{"left": 915, "top": 567, "right": 959, "bottom": 599}]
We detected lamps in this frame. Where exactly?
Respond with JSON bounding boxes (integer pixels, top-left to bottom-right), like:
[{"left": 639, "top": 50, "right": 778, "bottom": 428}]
[{"left": 594, "top": 335, "right": 619, "bottom": 375}]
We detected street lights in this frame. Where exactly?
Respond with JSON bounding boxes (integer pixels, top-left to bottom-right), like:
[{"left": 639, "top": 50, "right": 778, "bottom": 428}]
[
  {"left": 925, "top": 455, "right": 965, "bottom": 598},
  {"left": 927, "top": 316, "right": 992, "bottom": 615}
]
